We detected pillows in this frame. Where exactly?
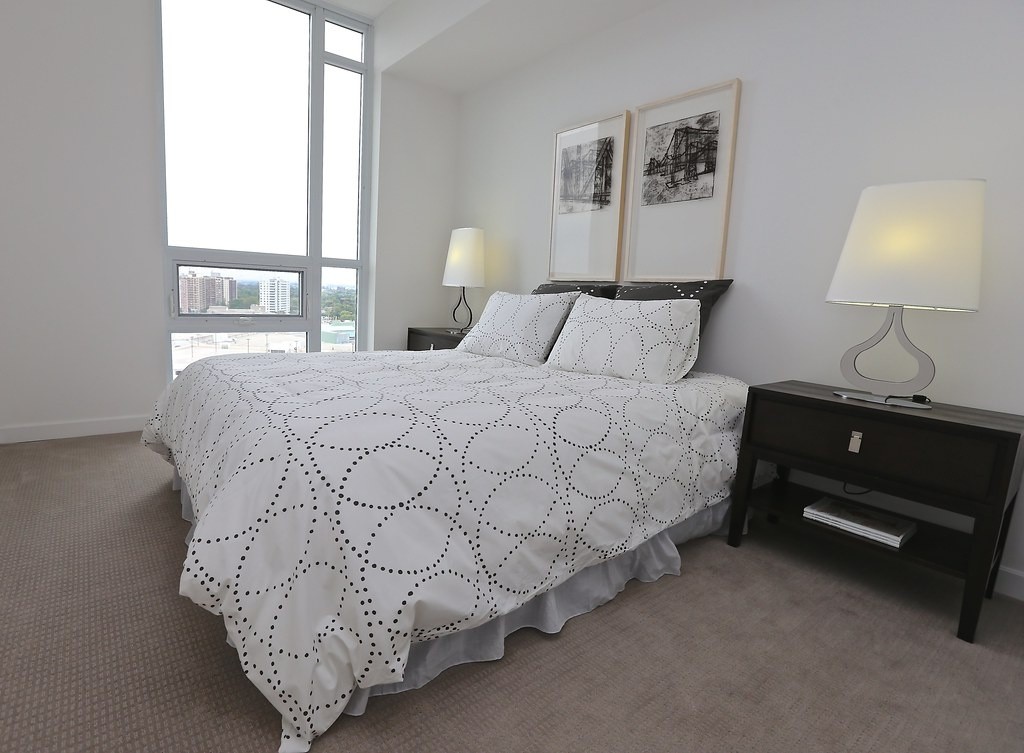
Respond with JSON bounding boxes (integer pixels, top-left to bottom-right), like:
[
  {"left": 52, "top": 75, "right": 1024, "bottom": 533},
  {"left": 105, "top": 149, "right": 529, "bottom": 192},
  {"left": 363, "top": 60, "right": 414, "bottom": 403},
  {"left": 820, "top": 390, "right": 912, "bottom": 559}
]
[
  {"left": 531, "top": 281, "right": 619, "bottom": 306},
  {"left": 544, "top": 293, "right": 704, "bottom": 384},
  {"left": 454, "top": 290, "right": 580, "bottom": 367},
  {"left": 611, "top": 279, "right": 731, "bottom": 337}
]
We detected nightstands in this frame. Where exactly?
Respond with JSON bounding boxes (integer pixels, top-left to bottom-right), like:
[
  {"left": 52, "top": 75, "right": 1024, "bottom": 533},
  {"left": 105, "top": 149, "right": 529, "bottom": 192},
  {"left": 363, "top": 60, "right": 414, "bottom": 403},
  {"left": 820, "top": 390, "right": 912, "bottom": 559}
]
[
  {"left": 726, "top": 376, "right": 1024, "bottom": 642},
  {"left": 406, "top": 324, "right": 476, "bottom": 353}
]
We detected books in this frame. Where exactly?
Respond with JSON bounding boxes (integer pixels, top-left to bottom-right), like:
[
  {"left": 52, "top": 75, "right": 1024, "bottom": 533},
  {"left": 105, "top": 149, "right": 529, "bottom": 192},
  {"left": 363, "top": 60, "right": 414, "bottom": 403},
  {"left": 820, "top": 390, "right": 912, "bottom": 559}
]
[{"left": 802, "top": 496, "right": 918, "bottom": 549}]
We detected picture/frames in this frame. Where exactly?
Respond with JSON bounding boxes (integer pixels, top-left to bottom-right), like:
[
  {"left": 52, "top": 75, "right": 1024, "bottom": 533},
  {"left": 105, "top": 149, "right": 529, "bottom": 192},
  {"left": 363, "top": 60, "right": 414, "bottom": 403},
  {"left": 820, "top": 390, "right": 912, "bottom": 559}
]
[
  {"left": 624, "top": 79, "right": 741, "bottom": 282},
  {"left": 546, "top": 110, "right": 631, "bottom": 284}
]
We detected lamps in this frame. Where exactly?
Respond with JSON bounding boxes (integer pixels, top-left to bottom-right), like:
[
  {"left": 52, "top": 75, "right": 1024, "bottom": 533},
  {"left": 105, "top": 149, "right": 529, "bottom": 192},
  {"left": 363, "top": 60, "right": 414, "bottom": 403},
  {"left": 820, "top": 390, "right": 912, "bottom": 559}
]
[
  {"left": 829, "top": 176, "right": 986, "bottom": 411},
  {"left": 443, "top": 228, "right": 490, "bottom": 335}
]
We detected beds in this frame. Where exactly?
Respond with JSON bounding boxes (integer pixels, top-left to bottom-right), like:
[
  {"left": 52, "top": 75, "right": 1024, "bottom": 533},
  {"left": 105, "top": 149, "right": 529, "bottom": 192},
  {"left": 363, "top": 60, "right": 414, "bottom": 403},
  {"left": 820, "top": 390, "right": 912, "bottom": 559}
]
[{"left": 143, "top": 340, "right": 751, "bottom": 753}]
